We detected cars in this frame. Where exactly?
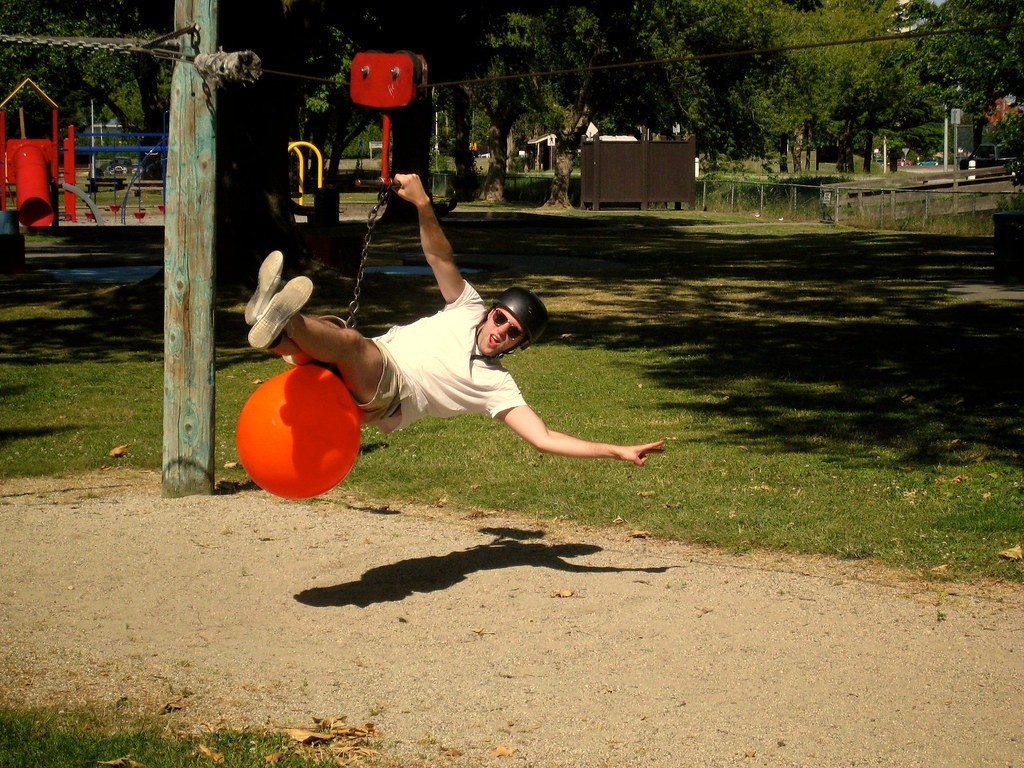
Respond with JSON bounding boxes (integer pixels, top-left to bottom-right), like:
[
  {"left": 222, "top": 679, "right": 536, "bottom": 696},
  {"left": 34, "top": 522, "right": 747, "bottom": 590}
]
[
  {"left": 876, "top": 159, "right": 884, "bottom": 166},
  {"left": 896, "top": 159, "right": 912, "bottom": 166}
]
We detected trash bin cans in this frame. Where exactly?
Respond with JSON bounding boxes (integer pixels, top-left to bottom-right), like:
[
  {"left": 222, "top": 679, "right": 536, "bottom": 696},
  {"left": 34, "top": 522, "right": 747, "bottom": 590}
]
[{"left": 993, "top": 209, "right": 1024, "bottom": 284}]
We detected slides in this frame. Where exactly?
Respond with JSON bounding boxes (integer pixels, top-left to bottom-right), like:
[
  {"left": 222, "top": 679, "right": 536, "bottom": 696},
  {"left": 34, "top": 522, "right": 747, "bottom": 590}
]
[{"left": 14, "top": 145, "right": 54, "bottom": 227}]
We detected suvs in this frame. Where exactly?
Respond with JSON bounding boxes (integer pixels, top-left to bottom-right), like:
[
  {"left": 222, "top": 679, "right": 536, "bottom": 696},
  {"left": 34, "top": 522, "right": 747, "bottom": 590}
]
[
  {"left": 959, "top": 142, "right": 1017, "bottom": 170},
  {"left": 110, "top": 156, "right": 132, "bottom": 176},
  {"left": 919, "top": 157, "right": 941, "bottom": 166}
]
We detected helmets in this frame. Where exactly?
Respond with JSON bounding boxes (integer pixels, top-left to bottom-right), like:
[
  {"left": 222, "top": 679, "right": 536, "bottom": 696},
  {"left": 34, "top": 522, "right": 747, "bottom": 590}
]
[{"left": 495, "top": 287, "right": 550, "bottom": 343}]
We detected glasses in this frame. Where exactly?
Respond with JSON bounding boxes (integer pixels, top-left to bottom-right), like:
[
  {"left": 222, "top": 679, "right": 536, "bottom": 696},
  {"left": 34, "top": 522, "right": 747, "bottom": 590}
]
[{"left": 492, "top": 309, "right": 523, "bottom": 341}]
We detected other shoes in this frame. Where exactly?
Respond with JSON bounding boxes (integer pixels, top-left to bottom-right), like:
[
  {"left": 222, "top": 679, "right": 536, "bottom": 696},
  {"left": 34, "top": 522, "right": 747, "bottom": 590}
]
[
  {"left": 245, "top": 250, "right": 285, "bottom": 325},
  {"left": 248, "top": 276, "right": 313, "bottom": 348}
]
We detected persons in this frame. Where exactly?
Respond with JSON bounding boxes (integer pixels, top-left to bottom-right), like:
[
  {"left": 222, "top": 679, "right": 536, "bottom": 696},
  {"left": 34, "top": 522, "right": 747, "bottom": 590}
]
[{"left": 244, "top": 173, "right": 669, "bottom": 467}]
